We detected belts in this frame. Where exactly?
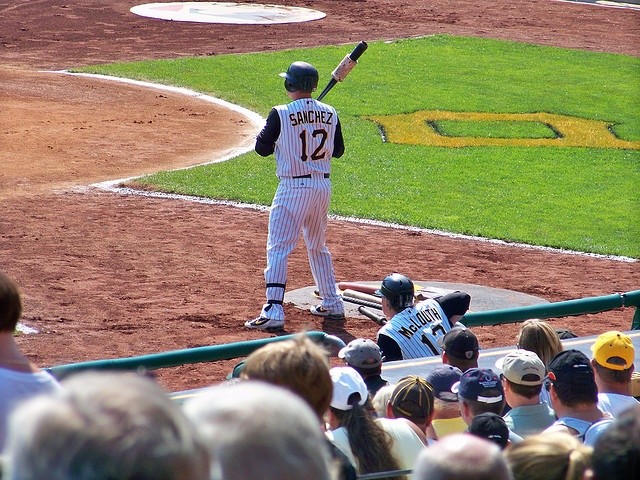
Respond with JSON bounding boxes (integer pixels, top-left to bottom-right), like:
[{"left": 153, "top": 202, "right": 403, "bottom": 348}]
[{"left": 294, "top": 174, "right": 330, "bottom": 179}]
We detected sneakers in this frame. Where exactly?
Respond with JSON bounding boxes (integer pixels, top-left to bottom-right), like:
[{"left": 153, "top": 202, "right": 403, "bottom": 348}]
[
  {"left": 309, "top": 305, "right": 346, "bottom": 321},
  {"left": 244, "top": 317, "right": 285, "bottom": 331}
]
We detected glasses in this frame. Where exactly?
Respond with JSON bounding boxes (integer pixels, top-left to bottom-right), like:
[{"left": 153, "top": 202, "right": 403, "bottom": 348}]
[{"left": 545, "top": 382, "right": 550, "bottom": 391}]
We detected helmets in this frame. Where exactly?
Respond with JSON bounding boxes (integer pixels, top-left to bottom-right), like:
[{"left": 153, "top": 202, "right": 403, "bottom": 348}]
[
  {"left": 278, "top": 61, "right": 319, "bottom": 92},
  {"left": 373, "top": 273, "right": 414, "bottom": 309}
]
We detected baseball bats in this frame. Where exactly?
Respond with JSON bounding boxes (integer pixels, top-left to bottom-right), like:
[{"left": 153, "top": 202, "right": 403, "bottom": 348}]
[
  {"left": 343, "top": 288, "right": 382, "bottom": 304},
  {"left": 338, "top": 282, "right": 378, "bottom": 293},
  {"left": 316, "top": 40, "right": 368, "bottom": 101}
]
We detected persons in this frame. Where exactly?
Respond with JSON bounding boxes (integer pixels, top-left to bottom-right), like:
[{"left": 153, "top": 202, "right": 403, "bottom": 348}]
[
  {"left": 375, "top": 273, "right": 470, "bottom": 362},
  {"left": 468, "top": 412, "right": 511, "bottom": 453},
  {"left": 425, "top": 363, "right": 469, "bottom": 440},
  {"left": 591, "top": 403, "right": 640, "bottom": 480},
  {"left": 386, "top": 375, "right": 440, "bottom": 447},
  {"left": 225, "top": 359, "right": 247, "bottom": 380},
  {"left": 436, "top": 327, "right": 480, "bottom": 372},
  {"left": 516, "top": 318, "right": 563, "bottom": 408},
  {"left": 450, "top": 367, "right": 525, "bottom": 446},
  {"left": 554, "top": 327, "right": 578, "bottom": 340},
  {"left": 0, "top": 270, "right": 59, "bottom": 455},
  {"left": 240, "top": 326, "right": 359, "bottom": 480},
  {"left": 502, "top": 431, "right": 594, "bottom": 479},
  {"left": 371, "top": 385, "right": 399, "bottom": 418},
  {"left": 590, "top": 329, "right": 640, "bottom": 418},
  {"left": 3, "top": 369, "right": 210, "bottom": 480},
  {"left": 338, "top": 338, "right": 389, "bottom": 400},
  {"left": 412, "top": 434, "right": 513, "bottom": 480},
  {"left": 540, "top": 349, "right": 617, "bottom": 451},
  {"left": 245, "top": 60, "right": 346, "bottom": 330},
  {"left": 322, "top": 367, "right": 429, "bottom": 480}
]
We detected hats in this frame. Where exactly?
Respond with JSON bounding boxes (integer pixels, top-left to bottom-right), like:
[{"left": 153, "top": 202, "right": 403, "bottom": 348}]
[
  {"left": 451, "top": 367, "right": 504, "bottom": 404},
  {"left": 337, "top": 338, "right": 386, "bottom": 368},
  {"left": 329, "top": 366, "right": 369, "bottom": 411},
  {"left": 590, "top": 330, "right": 635, "bottom": 370},
  {"left": 541, "top": 350, "right": 595, "bottom": 385},
  {"left": 225, "top": 359, "right": 253, "bottom": 382},
  {"left": 495, "top": 349, "right": 546, "bottom": 386},
  {"left": 425, "top": 364, "right": 463, "bottom": 403},
  {"left": 390, "top": 375, "right": 435, "bottom": 418},
  {"left": 464, "top": 412, "right": 509, "bottom": 450},
  {"left": 436, "top": 327, "right": 479, "bottom": 360}
]
